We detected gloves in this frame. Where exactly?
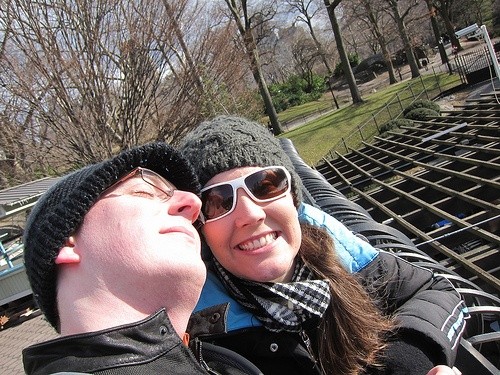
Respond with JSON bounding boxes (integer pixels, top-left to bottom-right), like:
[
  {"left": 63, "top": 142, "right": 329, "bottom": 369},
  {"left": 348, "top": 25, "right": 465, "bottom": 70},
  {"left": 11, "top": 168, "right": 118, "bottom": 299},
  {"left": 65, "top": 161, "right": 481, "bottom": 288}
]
[{"left": 355, "top": 327, "right": 448, "bottom": 375}]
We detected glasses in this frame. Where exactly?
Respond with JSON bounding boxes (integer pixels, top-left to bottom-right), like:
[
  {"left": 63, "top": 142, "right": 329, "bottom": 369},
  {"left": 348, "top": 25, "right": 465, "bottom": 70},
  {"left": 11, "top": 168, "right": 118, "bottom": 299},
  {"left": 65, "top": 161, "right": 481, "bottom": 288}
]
[
  {"left": 193, "top": 165, "right": 291, "bottom": 224},
  {"left": 94, "top": 166, "right": 206, "bottom": 231}
]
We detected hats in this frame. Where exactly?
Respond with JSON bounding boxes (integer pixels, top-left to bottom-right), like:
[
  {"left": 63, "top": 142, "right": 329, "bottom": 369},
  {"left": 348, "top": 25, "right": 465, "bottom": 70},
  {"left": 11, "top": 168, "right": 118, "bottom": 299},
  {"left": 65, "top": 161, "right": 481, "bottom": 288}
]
[
  {"left": 173, "top": 114, "right": 304, "bottom": 210},
  {"left": 23, "top": 139, "right": 203, "bottom": 335}
]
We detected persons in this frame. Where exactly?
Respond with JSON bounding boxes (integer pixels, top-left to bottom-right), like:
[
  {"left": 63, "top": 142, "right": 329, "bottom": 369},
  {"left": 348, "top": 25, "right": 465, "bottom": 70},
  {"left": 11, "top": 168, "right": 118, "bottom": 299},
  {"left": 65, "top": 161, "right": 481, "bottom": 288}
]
[
  {"left": 177, "top": 116, "right": 471, "bottom": 375},
  {"left": 21, "top": 142, "right": 322, "bottom": 375}
]
